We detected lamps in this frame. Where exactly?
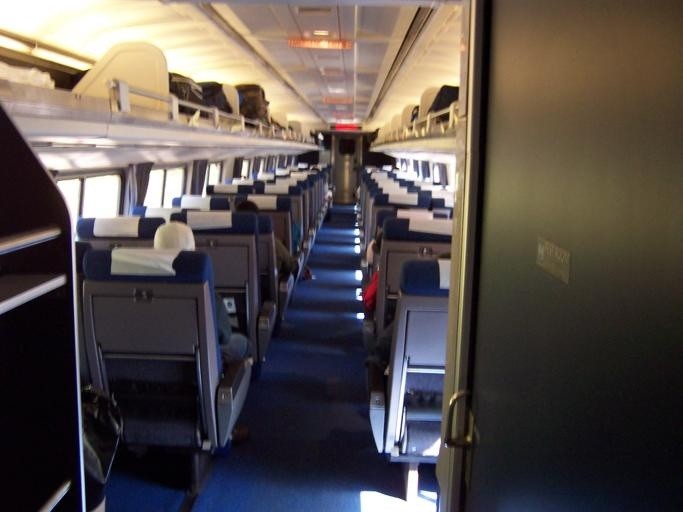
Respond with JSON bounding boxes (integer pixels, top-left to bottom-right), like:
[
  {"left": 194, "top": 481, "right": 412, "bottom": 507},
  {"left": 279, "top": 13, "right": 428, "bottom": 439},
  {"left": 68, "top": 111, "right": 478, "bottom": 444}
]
[{"left": 336, "top": 125, "right": 358, "bottom": 131}]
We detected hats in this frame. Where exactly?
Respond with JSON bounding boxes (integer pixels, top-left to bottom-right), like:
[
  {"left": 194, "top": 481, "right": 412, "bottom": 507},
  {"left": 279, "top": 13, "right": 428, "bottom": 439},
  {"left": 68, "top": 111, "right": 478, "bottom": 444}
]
[{"left": 154, "top": 222, "right": 194, "bottom": 251}]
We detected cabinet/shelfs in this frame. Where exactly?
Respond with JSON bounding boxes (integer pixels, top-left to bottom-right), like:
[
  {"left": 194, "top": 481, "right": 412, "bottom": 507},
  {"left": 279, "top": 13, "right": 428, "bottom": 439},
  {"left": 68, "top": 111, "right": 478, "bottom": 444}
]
[{"left": 0, "top": 101, "right": 88, "bottom": 512}]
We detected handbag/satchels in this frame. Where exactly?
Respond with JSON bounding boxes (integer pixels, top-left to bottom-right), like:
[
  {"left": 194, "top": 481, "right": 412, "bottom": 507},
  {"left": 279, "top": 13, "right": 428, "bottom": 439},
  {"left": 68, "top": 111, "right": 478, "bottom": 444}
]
[
  {"left": 428, "top": 83, "right": 460, "bottom": 121},
  {"left": 170, "top": 72, "right": 268, "bottom": 126}
]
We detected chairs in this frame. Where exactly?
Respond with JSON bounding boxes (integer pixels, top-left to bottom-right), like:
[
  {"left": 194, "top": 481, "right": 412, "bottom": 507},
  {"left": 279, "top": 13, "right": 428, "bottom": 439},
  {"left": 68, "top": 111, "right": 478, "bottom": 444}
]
[
  {"left": 354, "top": 164, "right": 458, "bottom": 505},
  {"left": 77, "top": 161, "right": 335, "bottom": 493}
]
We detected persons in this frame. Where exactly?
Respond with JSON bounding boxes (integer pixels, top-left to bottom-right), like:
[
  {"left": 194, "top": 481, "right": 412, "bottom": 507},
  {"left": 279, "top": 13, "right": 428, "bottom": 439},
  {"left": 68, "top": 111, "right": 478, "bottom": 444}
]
[
  {"left": 312, "top": 166, "right": 336, "bottom": 209},
  {"left": 362, "top": 231, "right": 383, "bottom": 315},
  {"left": 149, "top": 221, "right": 252, "bottom": 366},
  {"left": 233, "top": 200, "right": 296, "bottom": 341},
  {"left": 351, "top": 187, "right": 359, "bottom": 206},
  {"left": 361, "top": 318, "right": 392, "bottom": 367}
]
